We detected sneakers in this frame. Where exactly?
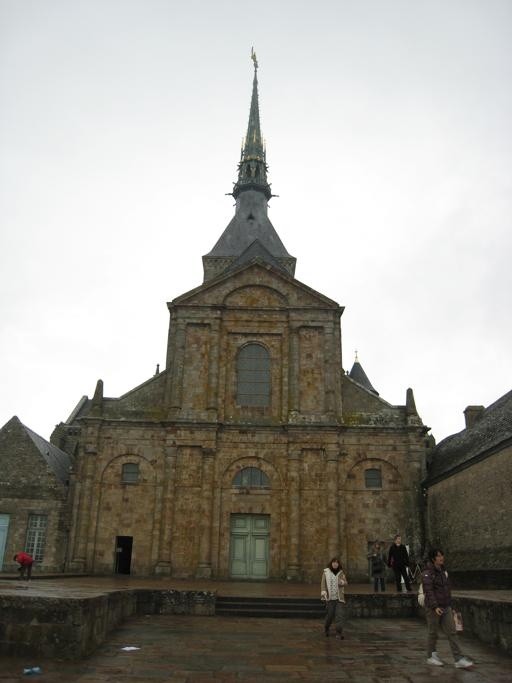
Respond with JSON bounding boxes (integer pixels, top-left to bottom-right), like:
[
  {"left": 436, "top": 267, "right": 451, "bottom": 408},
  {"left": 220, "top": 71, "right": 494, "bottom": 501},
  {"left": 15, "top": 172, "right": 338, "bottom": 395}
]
[{"left": 425, "top": 650, "right": 474, "bottom": 669}]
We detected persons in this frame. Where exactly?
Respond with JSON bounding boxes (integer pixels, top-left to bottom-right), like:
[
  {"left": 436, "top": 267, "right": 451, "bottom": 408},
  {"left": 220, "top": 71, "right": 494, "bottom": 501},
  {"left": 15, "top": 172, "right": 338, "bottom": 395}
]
[
  {"left": 421, "top": 547, "right": 474, "bottom": 670},
  {"left": 14, "top": 551, "right": 35, "bottom": 583},
  {"left": 366, "top": 540, "right": 389, "bottom": 591},
  {"left": 318, "top": 557, "right": 348, "bottom": 640},
  {"left": 387, "top": 535, "right": 413, "bottom": 591}
]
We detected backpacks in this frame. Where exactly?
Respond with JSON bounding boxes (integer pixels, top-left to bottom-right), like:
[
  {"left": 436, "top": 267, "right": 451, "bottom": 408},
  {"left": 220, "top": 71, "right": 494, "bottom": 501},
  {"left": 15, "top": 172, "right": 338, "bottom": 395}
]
[{"left": 415, "top": 568, "right": 449, "bottom": 607}]
